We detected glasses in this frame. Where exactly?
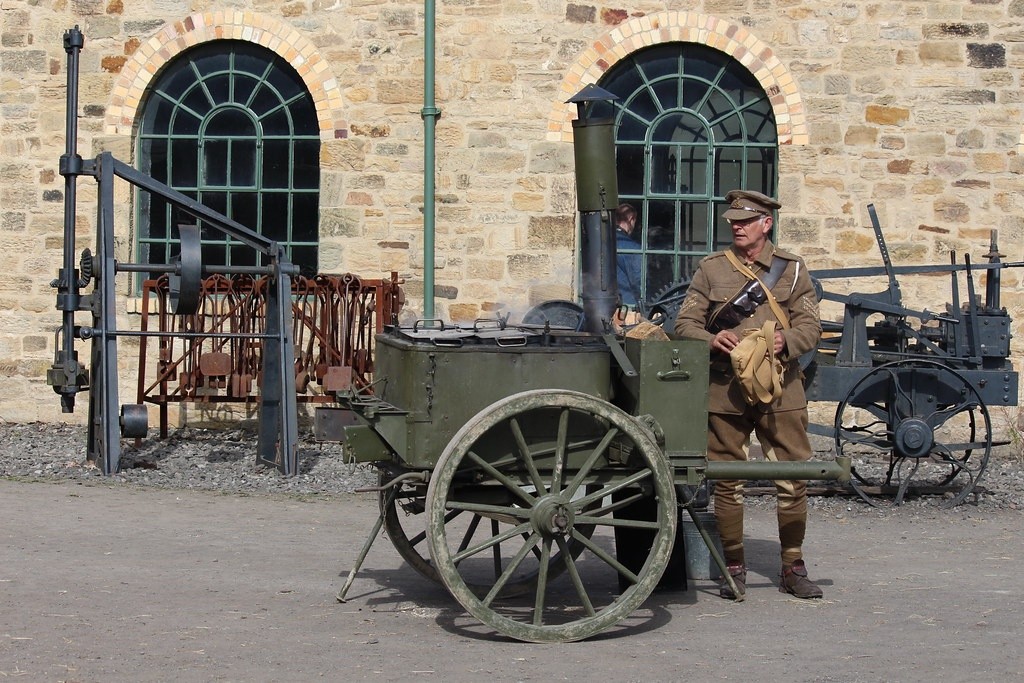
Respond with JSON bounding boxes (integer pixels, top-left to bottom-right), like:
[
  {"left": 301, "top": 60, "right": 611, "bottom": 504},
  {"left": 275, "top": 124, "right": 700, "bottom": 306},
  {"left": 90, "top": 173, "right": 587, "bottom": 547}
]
[{"left": 725, "top": 217, "right": 768, "bottom": 226}]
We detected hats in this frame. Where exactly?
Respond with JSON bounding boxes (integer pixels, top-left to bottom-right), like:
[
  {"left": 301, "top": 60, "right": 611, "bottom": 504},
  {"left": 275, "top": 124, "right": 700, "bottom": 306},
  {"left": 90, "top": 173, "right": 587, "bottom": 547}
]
[{"left": 721, "top": 189, "right": 782, "bottom": 221}]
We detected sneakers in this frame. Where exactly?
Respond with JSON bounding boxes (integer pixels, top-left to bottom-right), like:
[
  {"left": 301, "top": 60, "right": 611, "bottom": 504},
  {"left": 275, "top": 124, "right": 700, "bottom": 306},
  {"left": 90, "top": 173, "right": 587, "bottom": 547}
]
[
  {"left": 779, "top": 559, "right": 824, "bottom": 597},
  {"left": 719, "top": 561, "right": 748, "bottom": 598}
]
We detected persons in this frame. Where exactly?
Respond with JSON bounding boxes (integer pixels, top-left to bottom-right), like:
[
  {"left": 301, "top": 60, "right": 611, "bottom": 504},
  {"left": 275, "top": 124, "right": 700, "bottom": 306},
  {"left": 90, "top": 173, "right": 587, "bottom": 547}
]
[
  {"left": 615, "top": 204, "right": 641, "bottom": 304},
  {"left": 674, "top": 190, "right": 823, "bottom": 599}
]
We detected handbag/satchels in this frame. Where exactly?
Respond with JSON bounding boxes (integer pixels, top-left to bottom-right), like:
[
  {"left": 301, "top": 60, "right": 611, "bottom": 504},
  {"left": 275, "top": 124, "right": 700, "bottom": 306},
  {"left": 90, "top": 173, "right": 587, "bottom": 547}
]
[{"left": 729, "top": 320, "right": 788, "bottom": 406}]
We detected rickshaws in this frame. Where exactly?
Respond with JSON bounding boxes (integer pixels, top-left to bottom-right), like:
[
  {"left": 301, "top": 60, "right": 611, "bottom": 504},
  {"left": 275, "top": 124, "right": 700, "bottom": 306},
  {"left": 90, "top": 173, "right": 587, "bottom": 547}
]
[{"left": 314, "top": 83, "right": 851, "bottom": 644}]
